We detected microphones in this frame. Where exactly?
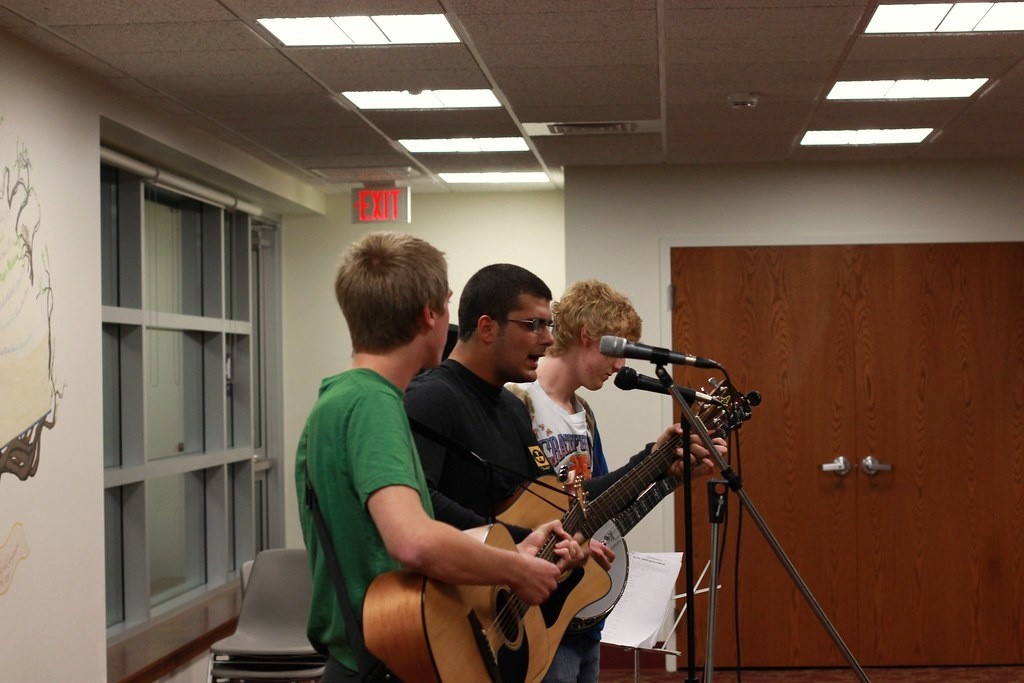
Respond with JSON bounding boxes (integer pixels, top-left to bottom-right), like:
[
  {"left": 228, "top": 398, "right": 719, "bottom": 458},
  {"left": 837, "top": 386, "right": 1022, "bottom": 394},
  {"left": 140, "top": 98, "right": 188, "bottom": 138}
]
[
  {"left": 613, "top": 366, "right": 724, "bottom": 406},
  {"left": 598, "top": 335, "right": 720, "bottom": 369}
]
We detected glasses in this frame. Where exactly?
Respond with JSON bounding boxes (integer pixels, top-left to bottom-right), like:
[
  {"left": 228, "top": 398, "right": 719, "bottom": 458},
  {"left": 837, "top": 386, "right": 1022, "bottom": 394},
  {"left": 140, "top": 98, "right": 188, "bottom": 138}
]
[{"left": 508, "top": 317, "right": 558, "bottom": 334}]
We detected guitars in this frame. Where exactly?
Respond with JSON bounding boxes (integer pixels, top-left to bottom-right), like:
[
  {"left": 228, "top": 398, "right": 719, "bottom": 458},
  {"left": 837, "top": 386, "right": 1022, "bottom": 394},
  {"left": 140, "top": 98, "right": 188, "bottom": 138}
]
[{"left": 362, "top": 373, "right": 763, "bottom": 683}]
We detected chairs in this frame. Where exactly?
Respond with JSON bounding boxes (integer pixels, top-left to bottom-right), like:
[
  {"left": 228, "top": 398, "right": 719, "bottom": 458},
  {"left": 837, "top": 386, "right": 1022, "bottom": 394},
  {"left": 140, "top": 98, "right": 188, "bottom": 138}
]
[{"left": 209, "top": 549, "right": 328, "bottom": 683}]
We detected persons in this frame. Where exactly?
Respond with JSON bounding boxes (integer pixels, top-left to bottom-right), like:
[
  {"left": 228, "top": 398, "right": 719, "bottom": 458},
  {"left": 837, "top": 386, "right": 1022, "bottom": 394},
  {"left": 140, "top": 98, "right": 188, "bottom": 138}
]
[
  {"left": 401, "top": 262, "right": 712, "bottom": 682},
  {"left": 293, "top": 230, "right": 584, "bottom": 683},
  {"left": 508, "top": 277, "right": 729, "bottom": 683}
]
